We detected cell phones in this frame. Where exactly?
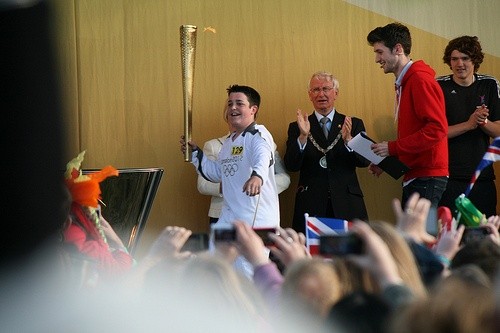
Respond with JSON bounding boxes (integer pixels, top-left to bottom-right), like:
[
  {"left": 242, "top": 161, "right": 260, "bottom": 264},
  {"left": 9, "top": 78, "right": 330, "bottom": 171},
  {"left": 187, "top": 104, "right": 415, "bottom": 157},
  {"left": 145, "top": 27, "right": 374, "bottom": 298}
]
[
  {"left": 319, "top": 234, "right": 364, "bottom": 255},
  {"left": 178, "top": 226, "right": 278, "bottom": 254},
  {"left": 460, "top": 227, "right": 488, "bottom": 245}
]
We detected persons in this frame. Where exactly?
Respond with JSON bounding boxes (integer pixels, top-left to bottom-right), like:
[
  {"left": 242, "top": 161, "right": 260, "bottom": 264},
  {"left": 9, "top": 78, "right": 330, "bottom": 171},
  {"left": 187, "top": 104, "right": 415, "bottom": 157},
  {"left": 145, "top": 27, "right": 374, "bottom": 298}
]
[
  {"left": 180, "top": 84, "right": 292, "bottom": 282},
  {"left": 0, "top": 168, "right": 500, "bottom": 333},
  {"left": 283, "top": 72, "right": 372, "bottom": 237},
  {"left": 436, "top": 35, "right": 500, "bottom": 227},
  {"left": 366, "top": 22, "right": 450, "bottom": 236}
]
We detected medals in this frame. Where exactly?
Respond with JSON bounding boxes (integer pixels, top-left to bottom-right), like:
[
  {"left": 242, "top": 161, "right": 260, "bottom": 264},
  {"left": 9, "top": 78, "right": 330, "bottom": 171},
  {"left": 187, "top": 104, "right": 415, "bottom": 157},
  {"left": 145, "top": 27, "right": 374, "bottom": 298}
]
[{"left": 320, "top": 155, "right": 328, "bottom": 168}]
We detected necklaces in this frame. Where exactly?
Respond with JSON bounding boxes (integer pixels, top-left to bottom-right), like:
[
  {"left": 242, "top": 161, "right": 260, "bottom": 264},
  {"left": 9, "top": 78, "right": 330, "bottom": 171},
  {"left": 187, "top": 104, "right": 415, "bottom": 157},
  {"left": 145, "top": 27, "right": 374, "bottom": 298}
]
[{"left": 472, "top": 75, "right": 475, "bottom": 83}]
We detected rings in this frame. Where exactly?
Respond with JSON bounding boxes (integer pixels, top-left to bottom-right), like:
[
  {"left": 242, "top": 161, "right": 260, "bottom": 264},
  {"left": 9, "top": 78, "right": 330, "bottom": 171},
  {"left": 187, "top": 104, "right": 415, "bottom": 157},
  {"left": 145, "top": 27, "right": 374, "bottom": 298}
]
[
  {"left": 286, "top": 237, "right": 293, "bottom": 244},
  {"left": 406, "top": 208, "right": 413, "bottom": 216}
]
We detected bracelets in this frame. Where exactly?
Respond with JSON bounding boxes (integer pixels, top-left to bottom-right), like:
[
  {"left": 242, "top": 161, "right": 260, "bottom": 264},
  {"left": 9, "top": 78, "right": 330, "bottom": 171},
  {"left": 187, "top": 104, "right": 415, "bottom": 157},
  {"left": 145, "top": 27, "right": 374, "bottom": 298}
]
[
  {"left": 479, "top": 118, "right": 487, "bottom": 128},
  {"left": 436, "top": 254, "right": 451, "bottom": 266}
]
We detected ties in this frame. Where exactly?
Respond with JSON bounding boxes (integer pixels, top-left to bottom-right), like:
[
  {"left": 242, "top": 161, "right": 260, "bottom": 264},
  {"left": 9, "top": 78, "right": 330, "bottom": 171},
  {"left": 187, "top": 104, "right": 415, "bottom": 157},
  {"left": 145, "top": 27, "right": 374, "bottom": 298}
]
[{"left": 321, "top": 117, "right": 330, "bottom": 139}]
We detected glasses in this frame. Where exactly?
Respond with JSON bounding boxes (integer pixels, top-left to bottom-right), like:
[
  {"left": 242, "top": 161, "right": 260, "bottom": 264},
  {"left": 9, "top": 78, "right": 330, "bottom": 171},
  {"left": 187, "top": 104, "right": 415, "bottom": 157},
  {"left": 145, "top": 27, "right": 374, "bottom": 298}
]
[{"left": 309, "top": 87, "right": 334, "bottom": 94}]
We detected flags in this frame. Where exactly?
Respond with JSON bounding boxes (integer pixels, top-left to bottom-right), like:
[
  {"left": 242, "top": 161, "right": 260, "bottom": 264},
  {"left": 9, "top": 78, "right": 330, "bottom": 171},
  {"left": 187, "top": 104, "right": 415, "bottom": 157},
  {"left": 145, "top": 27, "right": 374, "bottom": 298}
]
[
  {"left": 306, "top": 216, "right": 353, "bottom": 259},
  {"left": 464, "top": 136, "right": 500, "bottom": 198}
]
